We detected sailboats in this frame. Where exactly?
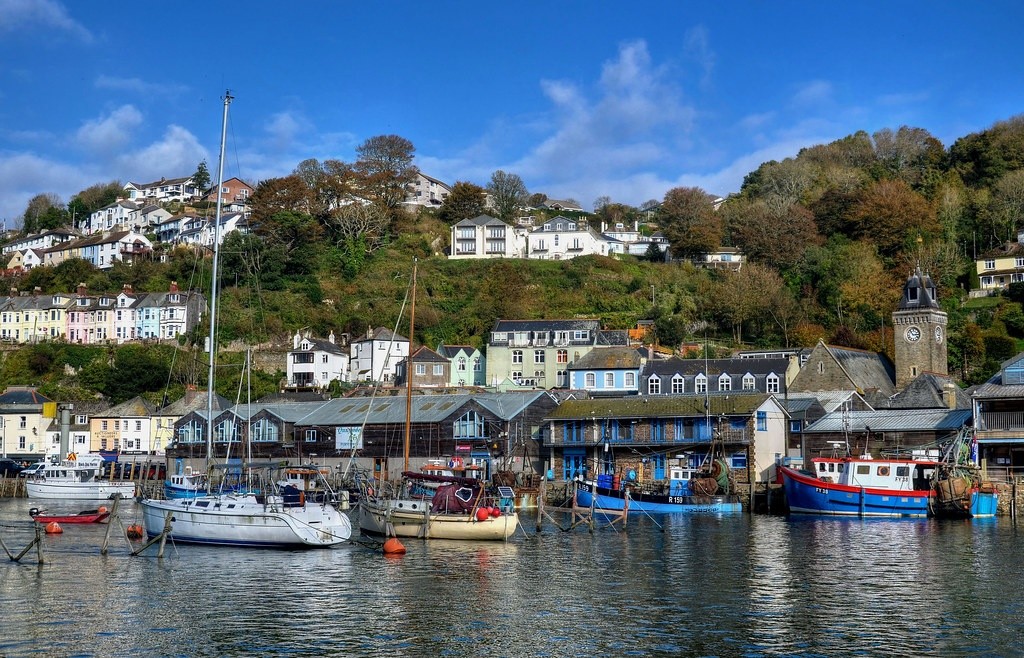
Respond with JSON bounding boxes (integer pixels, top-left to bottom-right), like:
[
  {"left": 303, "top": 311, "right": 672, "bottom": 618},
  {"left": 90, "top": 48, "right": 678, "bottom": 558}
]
[{"left": 141, "top": 90, "right": 518, "bottom": 547}]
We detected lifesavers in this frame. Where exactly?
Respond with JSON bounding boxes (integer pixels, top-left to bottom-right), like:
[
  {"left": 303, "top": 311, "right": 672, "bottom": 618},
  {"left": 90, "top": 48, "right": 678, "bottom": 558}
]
[{"left": 450, "top": 457, "right": 463, "bottom": 470}]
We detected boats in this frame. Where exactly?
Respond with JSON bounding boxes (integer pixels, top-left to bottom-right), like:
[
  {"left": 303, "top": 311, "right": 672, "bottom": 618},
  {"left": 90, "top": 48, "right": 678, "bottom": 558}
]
[
  {"left": 29, "top": 507, "right": 110, "bottom": 523},
  {"left": 779, "top": 399, "right": 1007, "bottom": 518},
  {"left": 25, "top": 454, "right": 135, "bottom": 500},
  {"left": 576, "top": 477, "right": 742, "bottom": 515}
]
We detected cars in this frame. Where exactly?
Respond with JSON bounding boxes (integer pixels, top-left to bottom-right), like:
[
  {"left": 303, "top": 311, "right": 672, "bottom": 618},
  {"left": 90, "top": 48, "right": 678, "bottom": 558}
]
[
  {"left": 106, "top": 462, "right": 166, "bottom": 480},
  {"left": 0, "top": 459, "right": 51, "bottom": 478}
]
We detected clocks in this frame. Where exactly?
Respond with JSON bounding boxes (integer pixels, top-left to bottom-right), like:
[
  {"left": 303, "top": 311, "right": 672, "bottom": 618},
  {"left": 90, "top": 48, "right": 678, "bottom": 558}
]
[
  {"left": 935, "top": 326, "right": 944, "bottom": 345},
  {"left": 904, "top": 325, "right": 922, "bottom": 344}
]
